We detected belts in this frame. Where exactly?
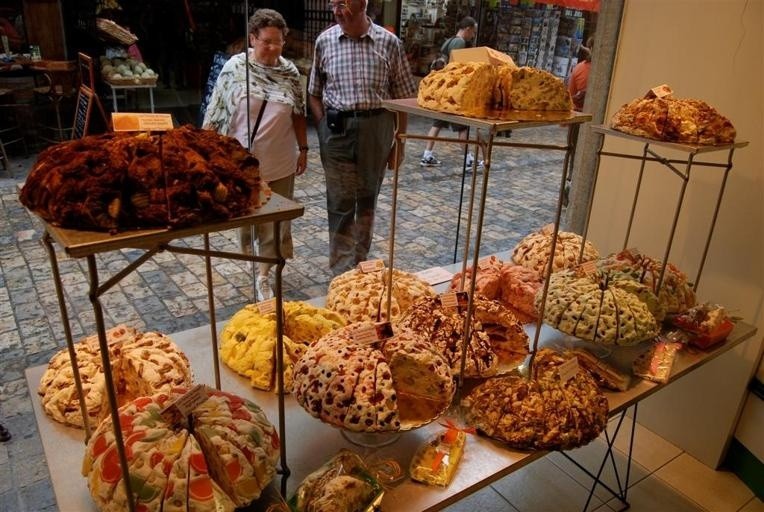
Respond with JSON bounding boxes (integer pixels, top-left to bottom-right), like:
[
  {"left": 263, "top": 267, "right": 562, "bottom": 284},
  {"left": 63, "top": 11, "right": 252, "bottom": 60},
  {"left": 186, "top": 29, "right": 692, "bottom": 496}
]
[{"left": 327, "top": 107, "right": 386, "bottom": 118}]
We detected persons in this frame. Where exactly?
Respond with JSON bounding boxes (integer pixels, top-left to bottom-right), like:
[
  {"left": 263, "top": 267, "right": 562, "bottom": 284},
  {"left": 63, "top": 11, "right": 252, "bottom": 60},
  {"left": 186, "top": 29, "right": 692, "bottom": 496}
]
[
  {"left": 419, "top": 16, "right": 485, "bottom": 172},
  {"left": 200, "top": 8, "right": 309, "bottom": 302},
  {"left": 307, "top": 0, "right": 418, "bottom": 279},
  {"left": 402, "top": 12, "right": 418, "bottom": 54},
  {"left": 561, "top": 36, "right": 595, "bottom": 208},
  {"left": 575, "top": 40, "right": 591, "bottom": 64}
]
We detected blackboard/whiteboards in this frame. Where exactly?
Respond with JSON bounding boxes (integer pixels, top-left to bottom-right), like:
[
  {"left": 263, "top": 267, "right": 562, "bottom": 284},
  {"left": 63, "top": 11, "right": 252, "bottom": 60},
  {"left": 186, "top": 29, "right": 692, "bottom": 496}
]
[
  {"left": 200, "top": 51, "right": 233, "bottom": 117},
  {"left": 71, "top": 86, "right": 93, "bottom": 141}
]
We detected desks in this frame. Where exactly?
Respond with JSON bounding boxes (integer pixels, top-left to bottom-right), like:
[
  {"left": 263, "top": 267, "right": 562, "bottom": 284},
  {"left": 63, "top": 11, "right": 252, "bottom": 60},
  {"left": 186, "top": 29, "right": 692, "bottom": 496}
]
[
  {"left": 102, "top": 80, "right": 158, "bottom": 112},
  {"left": 0, "top": 58, "right": 78, "bottom": 74}
]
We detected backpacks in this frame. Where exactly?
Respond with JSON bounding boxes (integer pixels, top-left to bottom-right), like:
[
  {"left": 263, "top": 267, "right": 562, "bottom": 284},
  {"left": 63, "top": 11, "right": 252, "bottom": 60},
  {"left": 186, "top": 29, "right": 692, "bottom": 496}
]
[{"left": 428, "top": 36, "right": 456, "bottom": 70}]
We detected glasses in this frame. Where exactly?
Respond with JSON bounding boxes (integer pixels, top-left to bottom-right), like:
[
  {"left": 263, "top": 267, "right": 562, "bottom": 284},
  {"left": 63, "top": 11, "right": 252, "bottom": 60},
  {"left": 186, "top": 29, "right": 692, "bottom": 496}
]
[
  {"left": 329, "top": 2, "right": 353, "bottom": 12},
  {"left": 254, "top": 34, "right": 288, "bottom": 46}
]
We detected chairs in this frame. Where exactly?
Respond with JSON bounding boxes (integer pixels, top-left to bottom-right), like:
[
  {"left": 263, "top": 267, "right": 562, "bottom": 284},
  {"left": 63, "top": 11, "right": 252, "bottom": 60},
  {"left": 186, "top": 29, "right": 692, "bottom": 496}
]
[
  {"left": 0, "top": 87, "right": 29, "bottom": 178},
  {"left": 32, "top": 71, "right": 84, "bottom": 152}
]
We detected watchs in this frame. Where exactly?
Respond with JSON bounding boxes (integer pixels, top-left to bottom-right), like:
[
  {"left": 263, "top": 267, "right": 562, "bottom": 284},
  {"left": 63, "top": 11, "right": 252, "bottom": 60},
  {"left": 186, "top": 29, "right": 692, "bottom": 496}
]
[
  {"left": 398, "top": 138, "right": 406, "bottom": 144},
  {"left": 299, "top": 145, "right": 309, "bottom": 152}
]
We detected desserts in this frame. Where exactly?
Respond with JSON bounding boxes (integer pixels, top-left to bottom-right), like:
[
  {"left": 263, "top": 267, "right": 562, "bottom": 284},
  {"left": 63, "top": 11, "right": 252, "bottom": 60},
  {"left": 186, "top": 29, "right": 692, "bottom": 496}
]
[
  {"left": 417, "top": 64, "right": 572, "bottom": 119},
  {"left": 20, "top": 127, "right": 263, "bottom": 231},
  {"left": 38, "top": 225, "right": 729, "bottom": 512},
  {"left": 610, "top": 97, "right": 737, "bottom": 146}
]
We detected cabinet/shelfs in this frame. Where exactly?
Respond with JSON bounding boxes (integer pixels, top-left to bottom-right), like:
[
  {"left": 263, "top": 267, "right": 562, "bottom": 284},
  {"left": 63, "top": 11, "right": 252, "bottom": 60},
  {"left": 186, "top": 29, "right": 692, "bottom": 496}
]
[{"left": 494, "top": 8, "right": 533, "bottom": 66}]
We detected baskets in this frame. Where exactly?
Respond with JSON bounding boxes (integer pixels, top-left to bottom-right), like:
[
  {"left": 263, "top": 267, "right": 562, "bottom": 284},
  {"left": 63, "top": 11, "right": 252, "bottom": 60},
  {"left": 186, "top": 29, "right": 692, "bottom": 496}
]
[
  {"left": 103, "top": 72, "right": 159, "bottom": 86},
  {"left": 97, "top": 17, "right": 139, "bottom": 46}
]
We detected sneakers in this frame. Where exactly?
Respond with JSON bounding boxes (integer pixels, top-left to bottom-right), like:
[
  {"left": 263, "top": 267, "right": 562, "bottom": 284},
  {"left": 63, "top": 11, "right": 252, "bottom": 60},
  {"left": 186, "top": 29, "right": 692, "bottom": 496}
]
[
  {"left": 245, "top": 249, "right": 260, "bottom": 274},
  {"left": 465, "top": 159, "right": 485, "bottom": 171},
  {"left": 421, "top": 154, "right": 442, "bottom": 167},
  {"left": 255, "top": 276, "right": 274, "bottom": 302}
]
